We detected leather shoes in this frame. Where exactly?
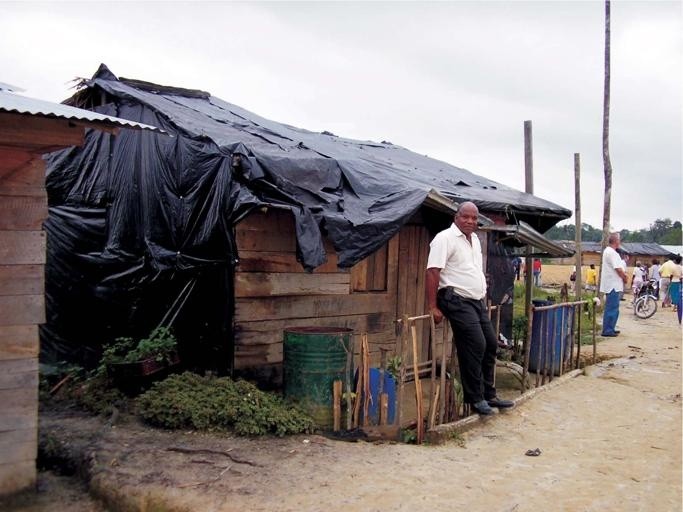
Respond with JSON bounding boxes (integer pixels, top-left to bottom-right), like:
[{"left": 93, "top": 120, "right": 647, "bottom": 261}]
[
  {"left": 471, "top": 397, "right": 514, "bottom": 415},
  {"left": 601, "top": 330, "right": 620, "bottom": 337}
]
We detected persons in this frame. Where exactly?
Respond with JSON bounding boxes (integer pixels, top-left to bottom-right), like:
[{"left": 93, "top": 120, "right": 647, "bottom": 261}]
[
  {"left": 426, "top": 201, "right": 514, "bottom": 415},
  {"left": 515, "top": 257, "right": 542, "bottom": 287},
  {"left": 570, "top": 264, "right": 596, "bottom": 296},
  {"left": 600, "top": 232, "right": 628, "bottom": 337},
  {"left": 621, "top": 254, "right": 683, "bottom": 324}
]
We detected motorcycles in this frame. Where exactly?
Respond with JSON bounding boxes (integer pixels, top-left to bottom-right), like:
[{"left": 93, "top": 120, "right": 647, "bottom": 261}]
[{"left": 632, "top": 280, "right": 658, "bottom": 318}]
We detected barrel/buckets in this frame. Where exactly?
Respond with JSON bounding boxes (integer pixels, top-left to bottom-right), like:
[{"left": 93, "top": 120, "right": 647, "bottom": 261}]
[
  {"left": 282, "top": 326, "right": 356, "bottom": 433},
  {"left": 523, "top": 297, "right": 574, "bottom": 374}
]
